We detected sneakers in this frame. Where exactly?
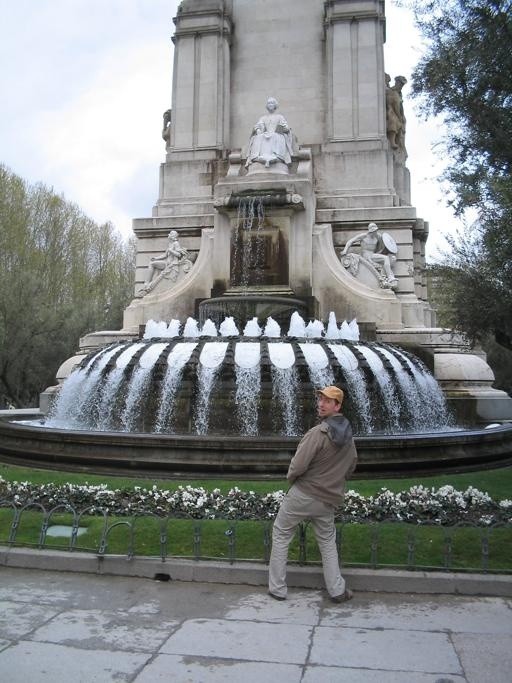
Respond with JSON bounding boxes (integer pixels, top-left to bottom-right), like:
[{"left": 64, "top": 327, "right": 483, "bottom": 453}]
[
  {"left": 332, "top": 588, "right": 353, "bottom": 603},
  {"left": 267, "top": 590, "right": 285, "bottom": 601}
]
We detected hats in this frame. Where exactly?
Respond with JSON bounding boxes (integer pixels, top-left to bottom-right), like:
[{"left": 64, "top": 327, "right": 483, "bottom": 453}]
[{"left": 315, "top": 385, "right": 344, "bottom": 403}]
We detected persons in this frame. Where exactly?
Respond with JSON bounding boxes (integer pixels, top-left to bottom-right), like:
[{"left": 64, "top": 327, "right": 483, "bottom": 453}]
[
  {"left": 385, "top": 73, "right": 407, "bottom": 148},
  {"left": 245, "top": 97, "right": 293, "bottom": 167},
  {"left": 139, "top": 231, "right": 182, "bottom": 290},
  {"left": 162, "top": 109, "right": 171, "bottom": 151},
  {"left": 340, "top": 223, "right": 398, "bottom": 283},
  {"left": 268, "top": 386, "right": 357, "bottom": 602}
]
